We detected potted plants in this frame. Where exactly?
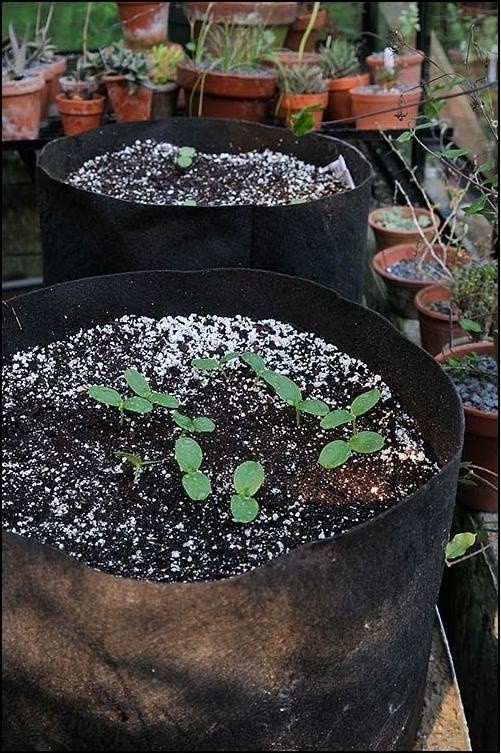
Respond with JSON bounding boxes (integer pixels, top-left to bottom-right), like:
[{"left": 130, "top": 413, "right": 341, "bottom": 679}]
[
  {"left": 372, "top": 66, "right": 498, "bottom": 471},
  {"left": 3, "top": 267, "right": 469, "bottom": 750},
  {"left": 33, "top": 114, "right": 372, "bottom": 308},
  {"left": 0, "top": 1, "right": 431, "bottom": 151}
]
